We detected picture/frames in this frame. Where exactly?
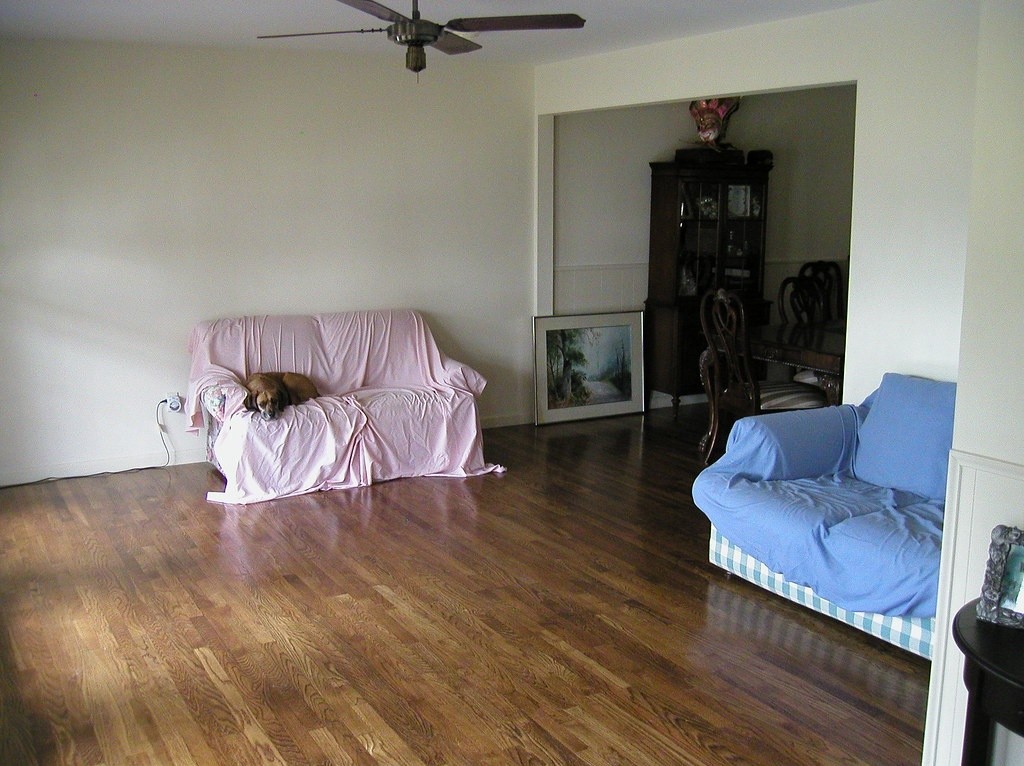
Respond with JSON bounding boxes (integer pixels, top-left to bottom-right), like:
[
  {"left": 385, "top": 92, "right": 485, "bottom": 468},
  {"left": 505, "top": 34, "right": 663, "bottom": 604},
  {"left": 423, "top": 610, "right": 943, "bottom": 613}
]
[
  {"left": 975, "top": 524, "right": 1024, "bottom": 630},
  {"left": 531, "top": 310, "right": 645, "bottom": 426}
]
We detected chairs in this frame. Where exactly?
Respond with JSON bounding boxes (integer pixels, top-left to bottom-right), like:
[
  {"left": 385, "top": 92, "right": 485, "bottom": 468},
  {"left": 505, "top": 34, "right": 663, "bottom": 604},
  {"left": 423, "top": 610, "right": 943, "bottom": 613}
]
[
  {"left": 696, "top": 285, "right": 833, "bottom": 466},
  {"left": 778, "top": 260, "right": 850, "bottom": 353}
]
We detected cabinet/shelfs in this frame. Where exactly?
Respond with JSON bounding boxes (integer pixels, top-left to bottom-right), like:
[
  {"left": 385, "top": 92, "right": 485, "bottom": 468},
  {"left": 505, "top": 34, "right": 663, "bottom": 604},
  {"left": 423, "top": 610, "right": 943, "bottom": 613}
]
[{"left": 644, "top": 145, "right": 775, "bottom": 420}]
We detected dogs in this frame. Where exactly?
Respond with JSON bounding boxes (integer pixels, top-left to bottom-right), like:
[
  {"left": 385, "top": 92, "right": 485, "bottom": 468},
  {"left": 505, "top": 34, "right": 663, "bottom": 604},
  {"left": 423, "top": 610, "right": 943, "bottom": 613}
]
[{"left": 243, "top": 372, "right": 322, "bottom": 422}]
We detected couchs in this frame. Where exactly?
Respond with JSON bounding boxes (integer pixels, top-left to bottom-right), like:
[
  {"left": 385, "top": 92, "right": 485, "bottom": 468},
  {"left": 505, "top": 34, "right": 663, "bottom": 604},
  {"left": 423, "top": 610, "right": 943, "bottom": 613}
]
[
  {"left": 184, "top": 307, "right": 506, "bottom": 505},
  {"left": 691, "top": 371, "right": 958, "bottom": 670}
]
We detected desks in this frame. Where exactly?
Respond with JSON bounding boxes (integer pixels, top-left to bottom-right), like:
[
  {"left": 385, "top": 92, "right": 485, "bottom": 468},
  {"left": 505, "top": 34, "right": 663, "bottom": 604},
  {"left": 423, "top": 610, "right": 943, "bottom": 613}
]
[
  {"left": 953, "top": 595, "right": 1024, "bottom": 766},
  {"left": 700, "top": 322, "right": 843, "bottom": 407}
]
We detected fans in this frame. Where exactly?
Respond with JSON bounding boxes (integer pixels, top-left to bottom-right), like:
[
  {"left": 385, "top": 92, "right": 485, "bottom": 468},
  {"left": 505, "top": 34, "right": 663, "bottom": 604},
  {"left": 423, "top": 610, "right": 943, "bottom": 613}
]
[{"left": 258, "top": 0, "right": 586, "bottom": 85}]
